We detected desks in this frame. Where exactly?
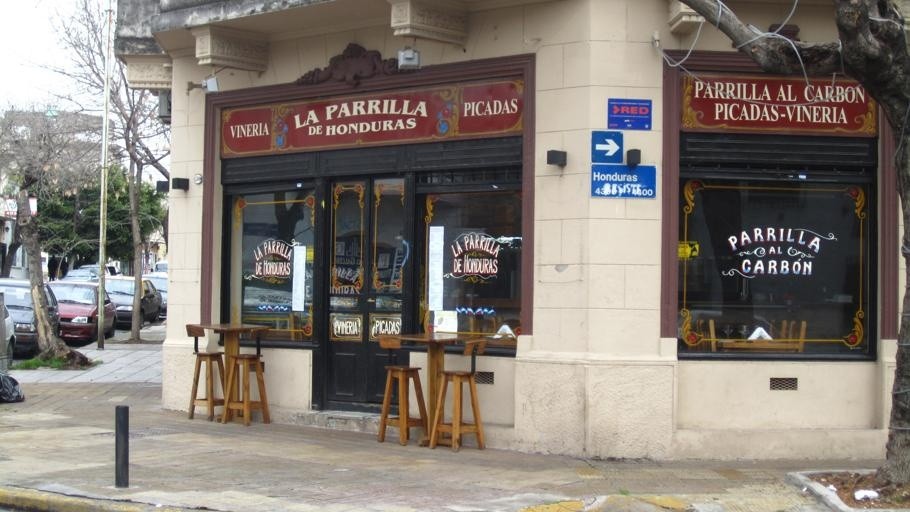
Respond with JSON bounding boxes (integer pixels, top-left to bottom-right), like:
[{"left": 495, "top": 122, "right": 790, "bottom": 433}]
[
  {"left": 379, "top": 331, "right": 487, "bottom": 446},
  {"left": 186, "top": 324, "right": 272, "bottom": 421}
]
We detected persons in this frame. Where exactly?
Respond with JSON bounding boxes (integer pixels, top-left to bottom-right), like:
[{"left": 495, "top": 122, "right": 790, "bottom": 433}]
[
  {"left": 60, "top": 258, "right": 68, "bottom": 279},
  {"left": 47, "top": 255, "right": 58, "bottom": 282}
]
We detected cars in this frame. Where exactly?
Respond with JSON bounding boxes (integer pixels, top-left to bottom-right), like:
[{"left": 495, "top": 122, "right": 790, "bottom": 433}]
[{"left": 0, "top": 261, "right": 168, "bottom": 367}]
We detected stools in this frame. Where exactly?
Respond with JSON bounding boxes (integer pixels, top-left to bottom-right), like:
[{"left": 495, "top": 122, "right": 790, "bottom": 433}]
[
  {"left": 376, "top": 365, "right": 487, "bottom": 452},
  {"left": 187, "top": 352, "right": 269, "bottom": 425}
]
